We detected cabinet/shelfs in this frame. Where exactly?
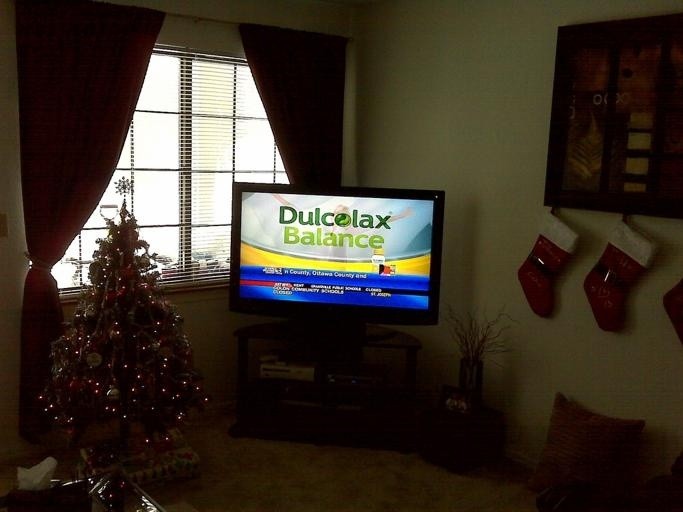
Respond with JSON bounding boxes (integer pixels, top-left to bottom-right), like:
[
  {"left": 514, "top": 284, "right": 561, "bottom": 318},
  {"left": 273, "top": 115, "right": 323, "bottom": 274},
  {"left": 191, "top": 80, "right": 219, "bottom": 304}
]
[{"left": 228, "top": 321, "right": 422, "bottom": 450}]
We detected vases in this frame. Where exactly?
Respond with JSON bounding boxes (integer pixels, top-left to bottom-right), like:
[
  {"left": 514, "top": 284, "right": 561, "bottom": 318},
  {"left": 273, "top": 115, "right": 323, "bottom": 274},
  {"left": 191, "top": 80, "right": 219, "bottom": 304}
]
[{"left": 458, "top": 357, "right": 484, "bottom": 400}]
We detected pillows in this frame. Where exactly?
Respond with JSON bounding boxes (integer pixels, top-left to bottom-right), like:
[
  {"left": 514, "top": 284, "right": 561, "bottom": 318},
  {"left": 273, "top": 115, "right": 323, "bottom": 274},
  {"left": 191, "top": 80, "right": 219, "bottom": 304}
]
[{"left": 527, "top": 391, "right": 646, "bottom": 510}]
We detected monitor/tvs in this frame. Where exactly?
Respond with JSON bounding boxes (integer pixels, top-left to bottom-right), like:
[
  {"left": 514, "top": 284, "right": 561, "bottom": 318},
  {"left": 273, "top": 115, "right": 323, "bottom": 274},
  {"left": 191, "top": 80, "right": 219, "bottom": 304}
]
[{"left": 227, "top": 181, "right": 445, "bottom": 349}]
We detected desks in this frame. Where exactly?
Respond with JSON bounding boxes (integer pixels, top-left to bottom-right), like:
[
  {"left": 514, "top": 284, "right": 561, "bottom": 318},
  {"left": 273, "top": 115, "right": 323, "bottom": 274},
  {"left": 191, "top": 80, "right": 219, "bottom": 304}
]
[{"left": 0, "top": 470, "right": 168, "bottom": 512}]
